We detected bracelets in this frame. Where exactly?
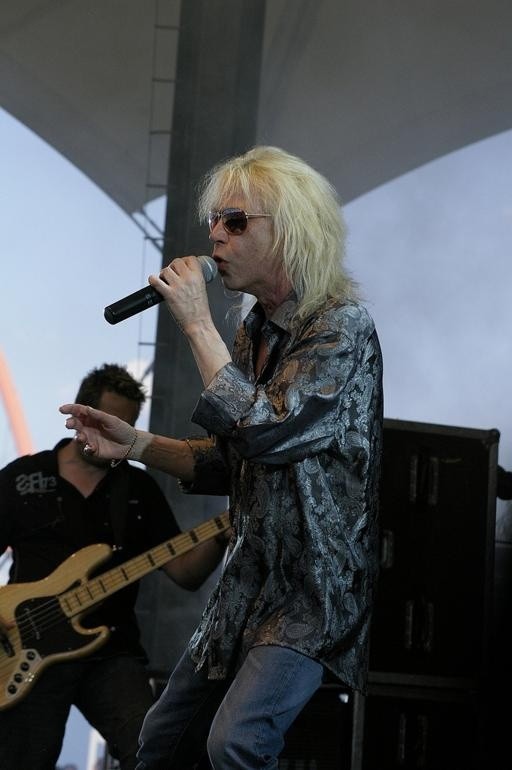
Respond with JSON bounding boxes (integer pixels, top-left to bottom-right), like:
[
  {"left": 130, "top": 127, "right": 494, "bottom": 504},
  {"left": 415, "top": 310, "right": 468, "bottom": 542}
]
[
  {"left": 110, "top": 429, "right": 138, "bottom": 468},
  {"left": 187, "top": 439, "right": 197, "bottom": 461}
]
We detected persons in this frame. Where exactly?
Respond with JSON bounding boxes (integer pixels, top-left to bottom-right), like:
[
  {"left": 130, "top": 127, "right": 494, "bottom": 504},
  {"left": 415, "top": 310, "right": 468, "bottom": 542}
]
[
  {"left": 1, "top": 364, "right": 232, "bottom": 769},
  {"left": 58, "top": 146, "right": 384, "bottom": 769}
]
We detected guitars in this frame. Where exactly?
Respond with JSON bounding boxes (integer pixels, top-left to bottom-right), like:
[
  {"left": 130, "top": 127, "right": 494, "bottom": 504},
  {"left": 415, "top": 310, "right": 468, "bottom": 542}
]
[{"left": 0, "top": 506, "right": 236, "bottom": 712}]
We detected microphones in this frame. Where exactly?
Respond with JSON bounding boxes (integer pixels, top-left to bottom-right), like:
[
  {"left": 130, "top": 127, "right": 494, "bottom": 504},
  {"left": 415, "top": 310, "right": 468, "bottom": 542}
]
[{"left": 104, "top": 255, "right": 218, "bottom": 324}]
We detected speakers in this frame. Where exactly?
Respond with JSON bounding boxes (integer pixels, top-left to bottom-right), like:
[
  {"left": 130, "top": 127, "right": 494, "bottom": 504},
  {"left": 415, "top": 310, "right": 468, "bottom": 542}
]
[
  {"left": 145, "top": 676, "right": 512, "bottom": 769},
  {"left": 366, "top": 417, "right": 499, "bottom": 688}
]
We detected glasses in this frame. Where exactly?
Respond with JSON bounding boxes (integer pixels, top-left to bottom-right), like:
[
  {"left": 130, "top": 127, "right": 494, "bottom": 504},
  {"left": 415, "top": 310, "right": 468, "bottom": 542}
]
[{"left": 207, "top": 205, "right": 275, "bottom": 236}]
